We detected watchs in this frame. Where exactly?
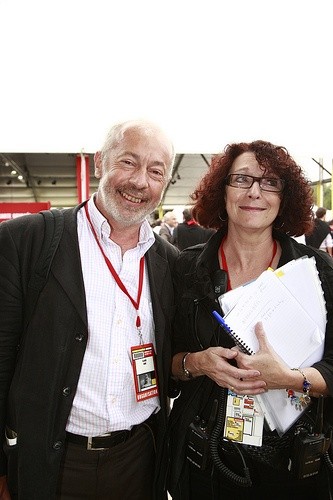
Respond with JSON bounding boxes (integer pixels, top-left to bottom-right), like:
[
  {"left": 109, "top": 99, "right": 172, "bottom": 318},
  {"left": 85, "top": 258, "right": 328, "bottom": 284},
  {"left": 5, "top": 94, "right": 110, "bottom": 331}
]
[{"left": 182, "top": 352, "right": 193, "bottom": 378}]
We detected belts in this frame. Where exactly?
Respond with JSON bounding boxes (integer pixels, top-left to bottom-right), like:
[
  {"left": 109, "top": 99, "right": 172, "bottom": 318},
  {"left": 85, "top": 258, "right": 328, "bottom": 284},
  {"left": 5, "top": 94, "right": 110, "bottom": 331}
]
[{"left": 65, "top": 409, "right": 163, "bottom": 450}]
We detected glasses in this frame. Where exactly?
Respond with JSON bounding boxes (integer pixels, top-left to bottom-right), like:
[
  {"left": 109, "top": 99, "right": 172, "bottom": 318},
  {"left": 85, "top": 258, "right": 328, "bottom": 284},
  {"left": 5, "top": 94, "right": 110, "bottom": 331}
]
[{"left": 225, "top": 173, "right": 286, "bottom": 193}]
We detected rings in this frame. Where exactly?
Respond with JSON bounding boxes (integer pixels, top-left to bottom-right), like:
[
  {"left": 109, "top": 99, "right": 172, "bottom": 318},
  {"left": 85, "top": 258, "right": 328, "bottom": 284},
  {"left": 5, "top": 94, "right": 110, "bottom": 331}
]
[{"left": 230, "top": 387, "right": 234, "bottom": 392}]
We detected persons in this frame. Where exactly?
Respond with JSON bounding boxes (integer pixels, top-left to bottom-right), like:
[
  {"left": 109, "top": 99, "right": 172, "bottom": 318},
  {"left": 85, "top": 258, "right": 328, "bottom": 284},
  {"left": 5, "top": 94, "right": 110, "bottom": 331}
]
[
  {"left": 150, "top": 207, "right": 333, "bottom": 259},
  {"left": 162, "top": 140, "right": 333, "bottom": 500},
  {"left": 0, "top": 119, "right": 181, "bottom": 500}
]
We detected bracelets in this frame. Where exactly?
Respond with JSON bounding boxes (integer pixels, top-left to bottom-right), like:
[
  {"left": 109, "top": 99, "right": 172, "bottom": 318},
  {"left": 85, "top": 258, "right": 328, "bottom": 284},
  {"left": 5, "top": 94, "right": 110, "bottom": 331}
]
[{"left": 286, "top": 368, "right": 311, "bottom": 411}]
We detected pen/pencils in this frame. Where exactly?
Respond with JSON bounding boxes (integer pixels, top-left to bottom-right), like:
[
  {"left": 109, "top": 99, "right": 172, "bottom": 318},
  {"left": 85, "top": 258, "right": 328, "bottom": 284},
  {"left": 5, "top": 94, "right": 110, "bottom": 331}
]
[{"left": 213, "top": 311, "right": 230, "bottom": 332}]
